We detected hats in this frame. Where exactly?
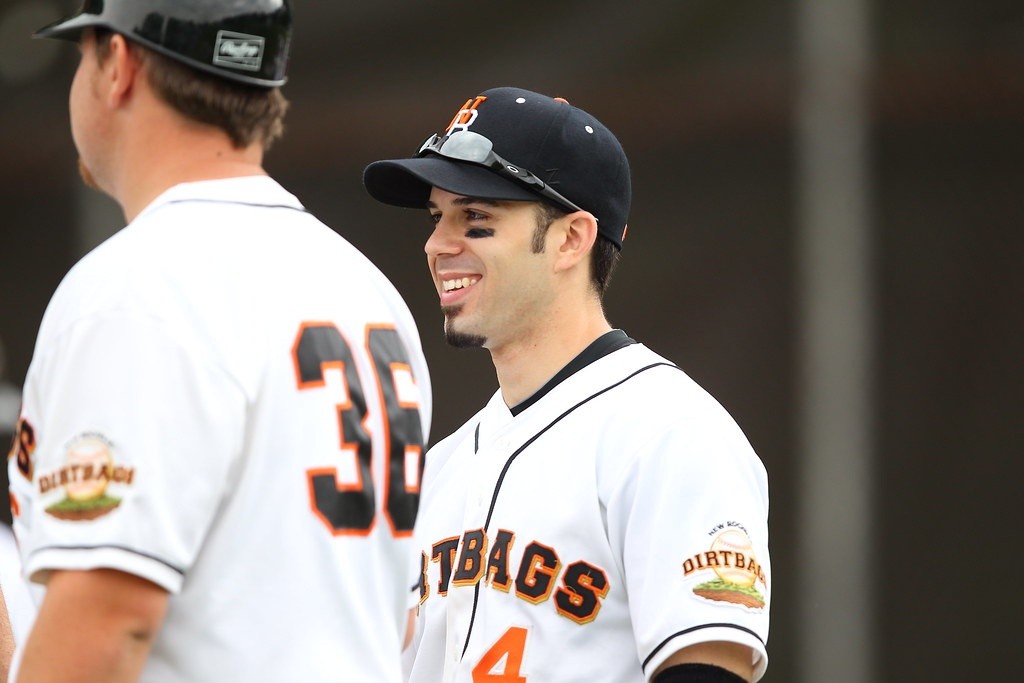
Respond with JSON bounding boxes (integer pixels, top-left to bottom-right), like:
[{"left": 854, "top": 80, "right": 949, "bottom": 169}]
[{"left": 363, "top": 85, "right": 632, "bottom": 251}]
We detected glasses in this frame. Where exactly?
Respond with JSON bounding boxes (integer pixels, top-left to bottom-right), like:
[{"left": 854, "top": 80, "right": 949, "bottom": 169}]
[{"left": 412, "top": 130, "right": 599, "bottom": 225}]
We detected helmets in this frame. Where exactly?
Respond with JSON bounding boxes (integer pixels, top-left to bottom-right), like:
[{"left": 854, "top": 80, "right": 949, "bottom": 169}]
[{"left": 30, "top": 0, "right": 291, "bottom": 85}]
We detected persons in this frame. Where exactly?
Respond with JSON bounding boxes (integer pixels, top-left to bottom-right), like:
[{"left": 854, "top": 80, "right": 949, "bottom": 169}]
[
  {"left": 363, "top": 85, "right": 771, "bottom": 683},
  {"left": 0, "top": 0, "right": 432, "bottom": 683}
]
